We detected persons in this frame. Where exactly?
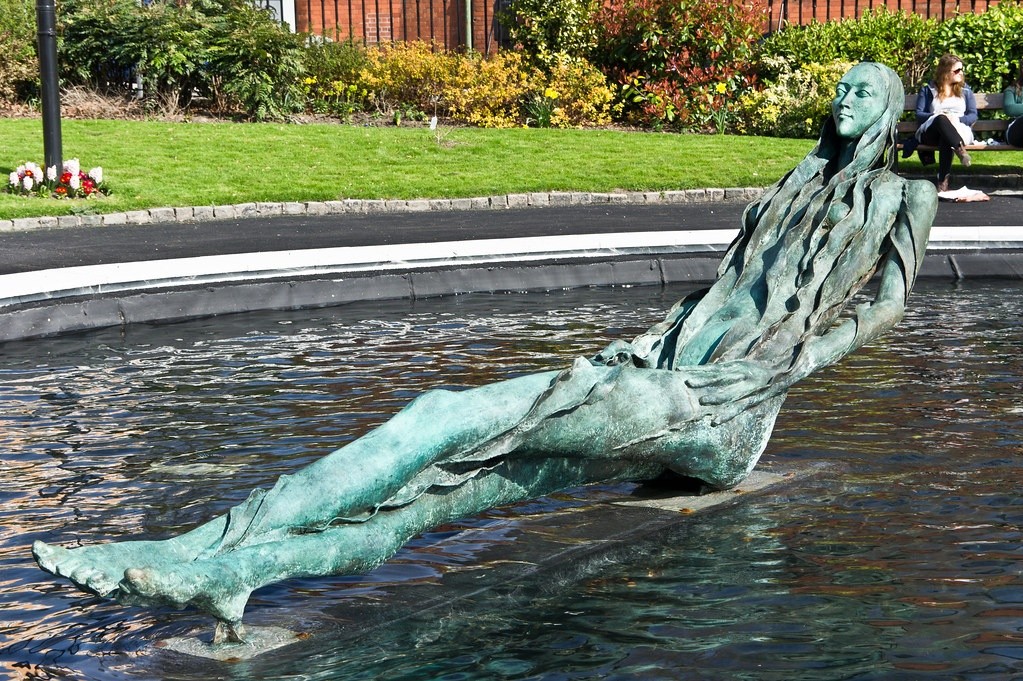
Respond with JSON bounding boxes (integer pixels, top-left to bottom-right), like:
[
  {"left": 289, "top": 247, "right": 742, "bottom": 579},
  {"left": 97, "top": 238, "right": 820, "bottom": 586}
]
[
  {"left": 1003, "top": 55, "right": 1023, "bottom": 147},
  {"left": 29, "top": 60, "right": 940, "bottom": 622},
  {"left": 914, "top": 55, "right": 977, "bottom": 192}
]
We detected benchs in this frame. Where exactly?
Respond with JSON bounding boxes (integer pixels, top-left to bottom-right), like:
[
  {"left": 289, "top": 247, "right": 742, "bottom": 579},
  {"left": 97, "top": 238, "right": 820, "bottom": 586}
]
[{"left": 894, "top": 93, "right": 1023, "bottom": 173}]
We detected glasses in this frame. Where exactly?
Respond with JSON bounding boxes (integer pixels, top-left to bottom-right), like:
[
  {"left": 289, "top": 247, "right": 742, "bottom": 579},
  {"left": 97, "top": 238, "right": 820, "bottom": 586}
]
[{"left": 949, "top": 68, "right": 963, "bottom": 74}]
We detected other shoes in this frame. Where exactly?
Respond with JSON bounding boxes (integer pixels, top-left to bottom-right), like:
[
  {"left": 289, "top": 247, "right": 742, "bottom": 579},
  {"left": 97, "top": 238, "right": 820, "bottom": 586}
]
[
  {"left": 936, "top": 173, "right": 949, "bottom": 192},
  {"left": 951, "top": 141, "right": 971, "bottom": 167}
]
